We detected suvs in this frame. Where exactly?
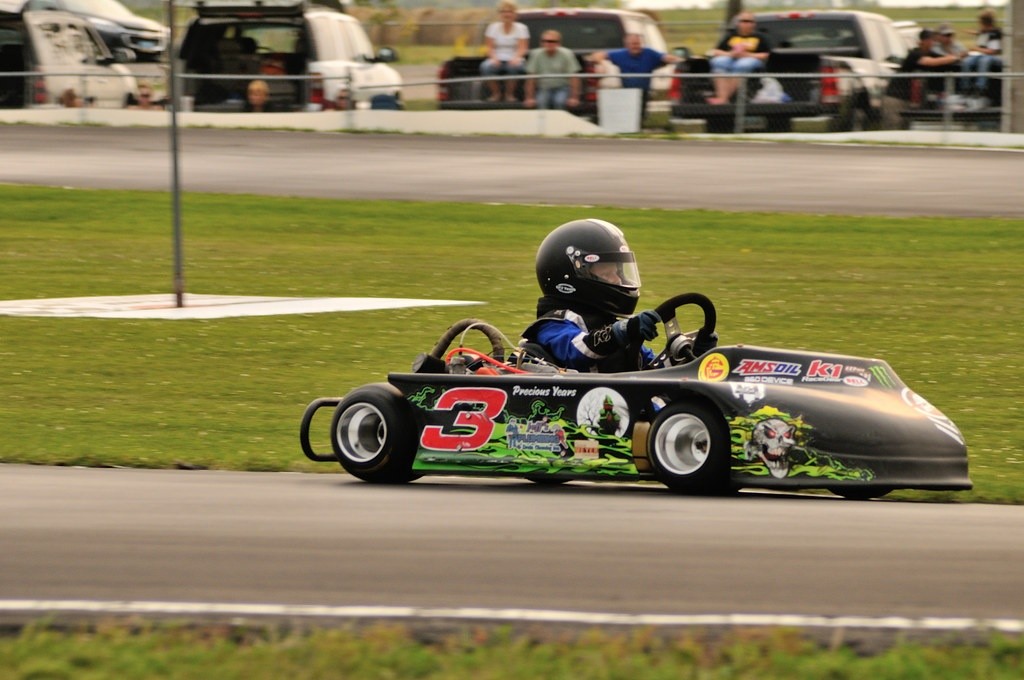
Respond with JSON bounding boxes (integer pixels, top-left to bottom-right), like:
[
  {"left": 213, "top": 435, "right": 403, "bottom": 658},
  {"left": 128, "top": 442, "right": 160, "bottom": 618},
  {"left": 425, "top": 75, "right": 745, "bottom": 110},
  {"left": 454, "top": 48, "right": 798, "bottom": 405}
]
[
  {"left": 18, "top": 2, "right": 171, "bottom": 60},
  {"left": 0, "top": 1, "right": 143, "bottom": 109},
  {"left": 165, "top": 0, "right": 404, "bottom": 112}
]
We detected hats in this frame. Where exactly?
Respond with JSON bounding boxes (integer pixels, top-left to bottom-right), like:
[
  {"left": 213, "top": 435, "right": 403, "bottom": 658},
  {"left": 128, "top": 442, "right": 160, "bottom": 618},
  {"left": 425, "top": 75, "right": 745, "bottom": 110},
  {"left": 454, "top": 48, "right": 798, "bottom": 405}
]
[
  {"left": 935, "top": 24, "right": 956, "bottom": 36},
  {"left": 918, "top": 29, "right": 939, "bottom": 40}
]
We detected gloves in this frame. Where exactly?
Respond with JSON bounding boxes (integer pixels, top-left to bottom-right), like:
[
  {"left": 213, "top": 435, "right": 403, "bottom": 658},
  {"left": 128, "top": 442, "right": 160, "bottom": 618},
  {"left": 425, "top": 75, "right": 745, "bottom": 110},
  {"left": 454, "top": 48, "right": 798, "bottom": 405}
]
[{"left": 632, "top": 310, "right": 661, "bottom": 342}]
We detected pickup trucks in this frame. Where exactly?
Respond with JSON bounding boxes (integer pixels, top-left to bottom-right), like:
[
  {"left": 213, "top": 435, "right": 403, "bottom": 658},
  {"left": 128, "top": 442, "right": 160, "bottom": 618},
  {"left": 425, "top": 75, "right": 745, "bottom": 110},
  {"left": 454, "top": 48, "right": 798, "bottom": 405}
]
[
  {"left": 438, "top": 6, "right": 692, "bottom": 114},
  {"left": 668, "top": 7, "right": 912, "bottom": 129}
]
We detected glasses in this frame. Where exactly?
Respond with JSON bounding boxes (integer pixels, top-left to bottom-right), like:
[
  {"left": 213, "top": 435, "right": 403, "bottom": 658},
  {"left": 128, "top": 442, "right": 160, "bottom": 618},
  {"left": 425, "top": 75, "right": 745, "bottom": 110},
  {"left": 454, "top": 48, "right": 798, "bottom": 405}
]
[
  {"left": 739, "top": 19, "right": 754, "bottom": 23},
  {"left": 542, "top": 39, "right": 560, "bottom": 43}
]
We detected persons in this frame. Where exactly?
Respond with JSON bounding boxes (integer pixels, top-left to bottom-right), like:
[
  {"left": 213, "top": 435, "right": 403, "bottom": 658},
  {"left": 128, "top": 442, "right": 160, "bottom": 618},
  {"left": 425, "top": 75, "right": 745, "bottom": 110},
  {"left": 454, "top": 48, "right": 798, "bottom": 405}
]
[
  {"left": 523, "top": 27, "right": 584, "bottom": 114},
  {"left": 512, "top": 212, "right": 669, "bottom": 373},
  {"left": 587, "top": 32, "right": 688, "bottom": 130},
  {"left": 885, "top": 28, "right": 959, "bottom": 109},
  {"left": 932, "top": 24, "right": 969, "bottom": 62},
  {"left": 236, "top": 79, "right": 284, "bottom": 115},
  {"left": 473, "top": 1, "right": 532, "bottom": 103},
  {"left": 960, "top": 8, "right": 1003, "bottom": 54},
  {"left": 944, "top": 46, "right": 1003, "bottom": 111},
  {"left": 703, "top": 9, "right": 771, "bottom": 107}
]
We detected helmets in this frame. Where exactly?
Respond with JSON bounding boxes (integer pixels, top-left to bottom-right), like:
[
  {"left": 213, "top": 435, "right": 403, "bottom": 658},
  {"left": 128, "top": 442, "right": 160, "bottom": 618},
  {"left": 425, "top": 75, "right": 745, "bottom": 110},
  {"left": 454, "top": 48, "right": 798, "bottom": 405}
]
[{"left": 535, "top": 216, "right": 641, "bottom": 319}]
